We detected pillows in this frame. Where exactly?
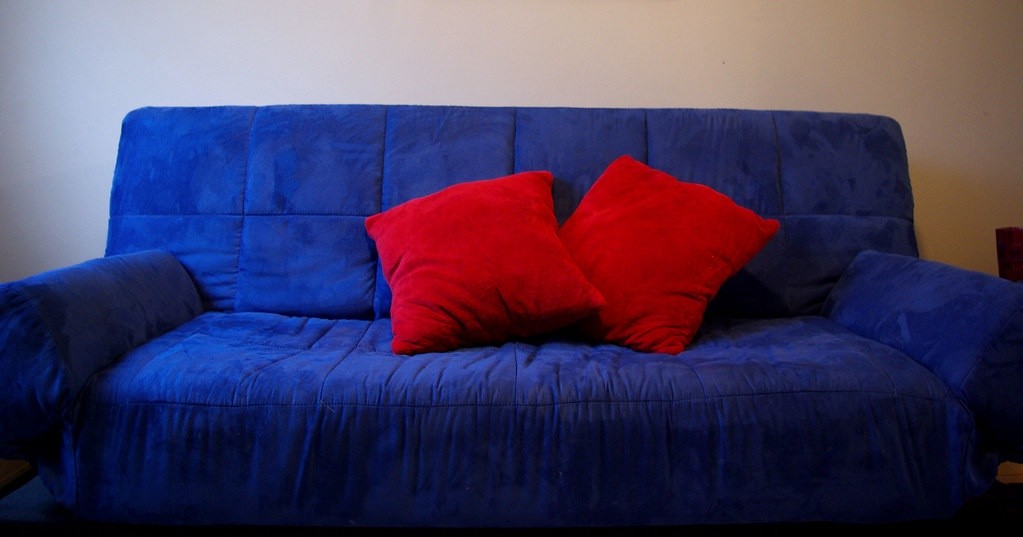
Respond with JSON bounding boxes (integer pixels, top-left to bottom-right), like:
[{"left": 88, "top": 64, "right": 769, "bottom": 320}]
[
  {"left": 365, "top": 171, "right": 605, "bottom": 355},
  {"left": 559, "top": 155, "right": 781, "bottom": 358}
]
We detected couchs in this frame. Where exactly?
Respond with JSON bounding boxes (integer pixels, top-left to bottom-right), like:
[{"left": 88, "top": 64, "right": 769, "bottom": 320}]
[{"left": 1, "top": 108, "right": 1023, "bottom": 537}]
